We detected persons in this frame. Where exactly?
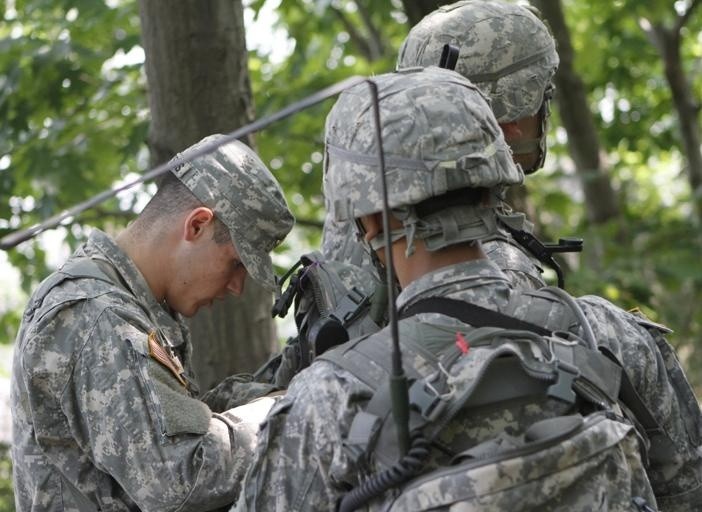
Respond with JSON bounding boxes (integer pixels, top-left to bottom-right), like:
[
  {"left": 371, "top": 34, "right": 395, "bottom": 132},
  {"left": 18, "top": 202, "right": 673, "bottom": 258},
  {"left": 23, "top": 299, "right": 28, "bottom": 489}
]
[
  {"left": 9, "top": 133, "right": 300, "bottom": 511},
  {"left": 222, "top": 64, "right": 702, "bottom": 511},
  {"left": 260, "top": 0, "right": 561, "bottom": 387}
]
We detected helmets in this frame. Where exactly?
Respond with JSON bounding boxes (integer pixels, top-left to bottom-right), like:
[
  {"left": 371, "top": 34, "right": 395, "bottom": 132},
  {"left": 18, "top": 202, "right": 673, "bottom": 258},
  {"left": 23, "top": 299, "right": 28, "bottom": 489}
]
[
  {"left": 396, "top": 0, "right": 560, "bottom": 126},
  {"left": 321, "top": 65, "right": 524, "bottom": 224}
]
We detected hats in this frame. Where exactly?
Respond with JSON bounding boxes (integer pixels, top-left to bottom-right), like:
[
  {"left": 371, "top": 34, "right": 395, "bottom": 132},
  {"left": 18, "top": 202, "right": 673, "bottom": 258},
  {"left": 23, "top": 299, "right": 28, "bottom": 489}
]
[{"left": 168, "top": 133, "right": 294, "bottom": 300}]
